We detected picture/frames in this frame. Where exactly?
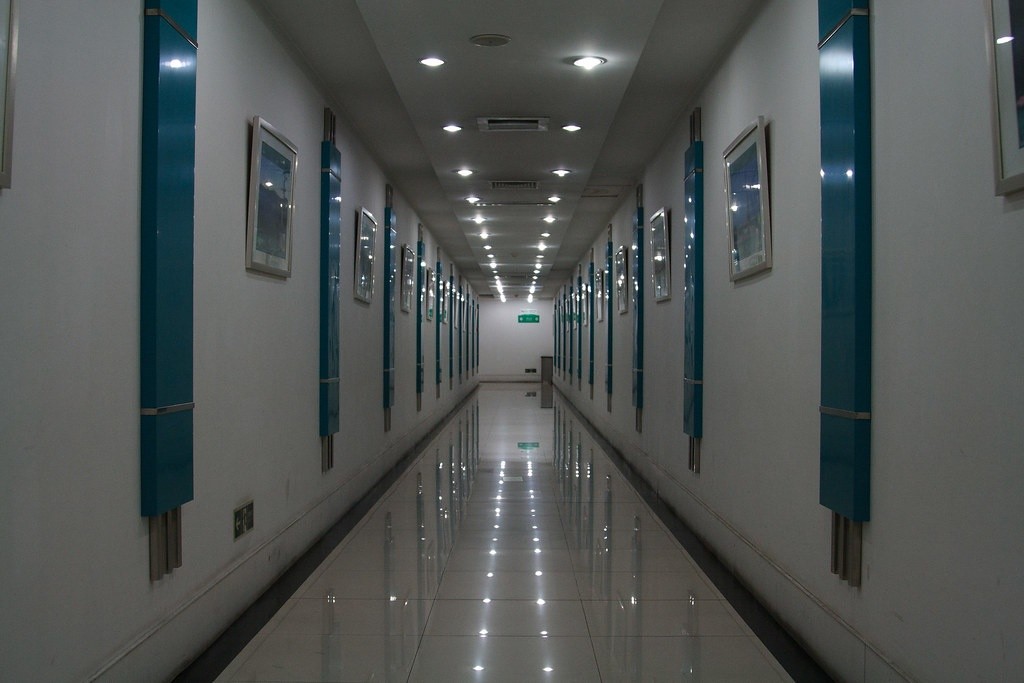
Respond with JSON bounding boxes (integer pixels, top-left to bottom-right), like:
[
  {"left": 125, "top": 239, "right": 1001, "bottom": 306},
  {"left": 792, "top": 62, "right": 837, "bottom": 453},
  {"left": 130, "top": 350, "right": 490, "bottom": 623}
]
[
  {"left": 616, "top": 245, "right": 629, "bottom": 315},
  {"left": 722, "top": 115, "right": 772, "bottom": 282},
  {"left": 559, "top": 268, "right": 604, "bottom": 334},
  {"left": 353, "top": 206, "right": 378, "bottom": 304},
  {"left": 426, "top": 267, "right": 476, "bottom": 333},
  {"left": 244, "top": 114, "right": 299, "bottom": 278},
  {"left": 400, "top": 244, "right": 416, "bottom": 313},
  {"left": 649, "top": 205, "right": 671, "bottom": 303}
]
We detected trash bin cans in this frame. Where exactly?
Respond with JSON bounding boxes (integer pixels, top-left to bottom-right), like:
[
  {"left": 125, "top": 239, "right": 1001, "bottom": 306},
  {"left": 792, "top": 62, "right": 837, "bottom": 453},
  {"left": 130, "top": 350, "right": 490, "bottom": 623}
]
[{"left": 540, "top": 355, "right": 553, "bottom": 384}]
